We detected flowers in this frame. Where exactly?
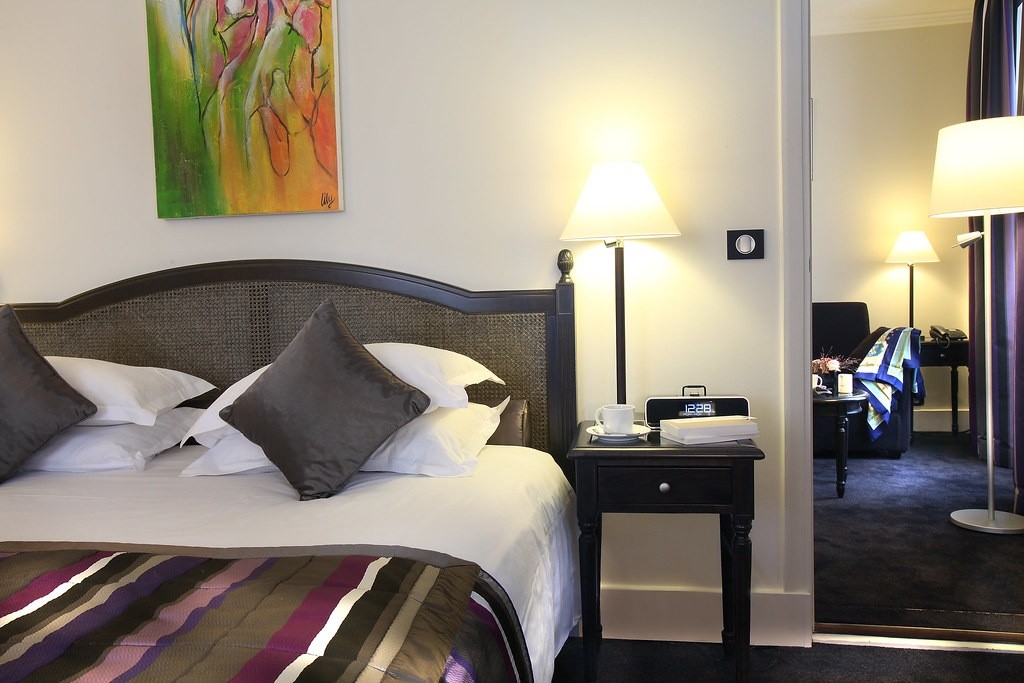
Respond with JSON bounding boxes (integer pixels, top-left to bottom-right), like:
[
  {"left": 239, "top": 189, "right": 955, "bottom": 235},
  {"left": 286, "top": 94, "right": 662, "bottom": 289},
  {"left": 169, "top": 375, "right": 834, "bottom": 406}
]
[{"left": 810, "top": 346, "right": 860, "bottom": 373}]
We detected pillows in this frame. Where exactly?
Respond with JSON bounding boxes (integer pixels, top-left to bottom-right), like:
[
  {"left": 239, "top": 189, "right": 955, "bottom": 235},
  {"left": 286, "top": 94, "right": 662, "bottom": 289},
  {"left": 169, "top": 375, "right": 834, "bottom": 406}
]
[
  {"left": 179, "top": 343, "right": 488, "bottom": 448},
  {"left": 180, "top": 396, "right": 513, "bottom": 480},
  {"left": 214, "top": 294, "right": 430, "bottom": 502},
  {"left": 27, "top": 409, "right": 207, "bottom": 471},
  {"left": 0, "top": 304, "right": 95, "bottom": 477},
  {"left": 39, "top": 353, "right": 219, "bottom": 425}
]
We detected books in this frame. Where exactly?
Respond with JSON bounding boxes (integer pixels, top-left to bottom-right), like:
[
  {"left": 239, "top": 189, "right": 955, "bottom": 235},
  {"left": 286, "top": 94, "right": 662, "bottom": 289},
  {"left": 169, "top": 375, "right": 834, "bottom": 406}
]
[{"left": 659, "top": 415, "right": 759, "bottom": 445}]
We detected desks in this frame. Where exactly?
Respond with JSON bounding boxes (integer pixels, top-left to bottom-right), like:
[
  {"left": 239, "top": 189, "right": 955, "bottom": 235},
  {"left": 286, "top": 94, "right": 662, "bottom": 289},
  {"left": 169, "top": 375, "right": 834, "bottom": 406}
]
[
  {"left": 814, "top": 390, "right": 871, "bottom": 498},
  {"left": 913, "top": 339, "right": 969, "bottom": 437},
  {"left": 566, "top": 417, "right": 767, "bottom": 682}
]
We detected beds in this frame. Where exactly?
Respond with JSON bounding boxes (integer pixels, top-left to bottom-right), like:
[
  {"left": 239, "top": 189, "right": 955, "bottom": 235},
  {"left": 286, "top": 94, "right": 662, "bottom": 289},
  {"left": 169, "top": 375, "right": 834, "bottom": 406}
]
[{"left": 0, "top": 247, "right": 581, "bottom": 683}]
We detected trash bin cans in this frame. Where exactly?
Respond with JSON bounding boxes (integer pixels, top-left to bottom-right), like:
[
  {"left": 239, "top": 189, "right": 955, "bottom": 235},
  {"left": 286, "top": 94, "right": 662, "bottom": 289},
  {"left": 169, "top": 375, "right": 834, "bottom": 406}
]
[{"left": 929, "top": 325, "right": 967, "bottom": 340}]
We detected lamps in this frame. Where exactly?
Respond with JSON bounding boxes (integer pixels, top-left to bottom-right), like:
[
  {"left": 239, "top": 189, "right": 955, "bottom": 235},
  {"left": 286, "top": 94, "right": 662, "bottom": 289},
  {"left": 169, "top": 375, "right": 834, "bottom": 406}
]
[
  {"left": 957, "top": 231, "right": 983, "bottom": 248},
  {"left": 935, "top": 112, "right": 1023, "bottom": 532},
  {"left": 883, "top": 231, "right": 940, "bottom": 328},
  {"left": 561, "top": 158, "right": 682, "bottom": 404}
]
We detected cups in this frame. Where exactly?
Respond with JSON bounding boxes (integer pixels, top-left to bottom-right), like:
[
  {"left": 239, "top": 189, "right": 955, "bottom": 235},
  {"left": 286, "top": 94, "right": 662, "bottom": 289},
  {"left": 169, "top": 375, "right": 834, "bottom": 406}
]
[
  {"left": 595, "top": 404, "right": 635, "bottom": 434},
  {"left": 837, "top": 374, "right": 853, "bottom": 394},
  {"left": 812, "top": 374, "right": 823, "bottom": 392}
]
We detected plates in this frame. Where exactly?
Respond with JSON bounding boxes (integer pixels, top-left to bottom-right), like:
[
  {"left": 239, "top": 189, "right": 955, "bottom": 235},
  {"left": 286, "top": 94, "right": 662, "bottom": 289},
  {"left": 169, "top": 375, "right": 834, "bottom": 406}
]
[{"left": 585, "top": 424, "right": 652, "bottom": 442}]
